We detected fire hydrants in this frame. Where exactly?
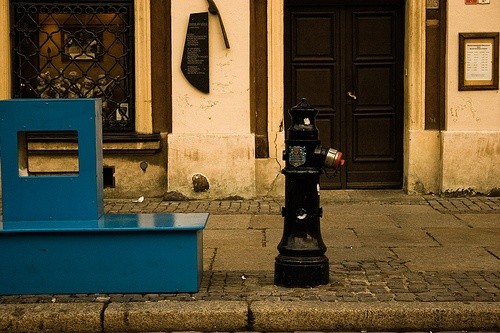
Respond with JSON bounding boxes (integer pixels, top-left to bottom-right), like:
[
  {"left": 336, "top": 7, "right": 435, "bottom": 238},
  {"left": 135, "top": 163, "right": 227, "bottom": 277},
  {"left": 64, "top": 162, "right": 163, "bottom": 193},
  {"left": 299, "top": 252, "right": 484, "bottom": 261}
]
[{"left": 274, "top": 98, "right": 346, "bottom": 288}]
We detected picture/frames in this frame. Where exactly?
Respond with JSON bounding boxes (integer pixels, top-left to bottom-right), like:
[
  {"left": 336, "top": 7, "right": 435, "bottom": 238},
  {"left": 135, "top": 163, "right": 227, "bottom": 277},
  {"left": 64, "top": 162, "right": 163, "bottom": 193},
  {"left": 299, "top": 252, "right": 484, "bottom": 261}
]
[{"left": 458, "top": 31, "right": 499, "bottom": 92}]
